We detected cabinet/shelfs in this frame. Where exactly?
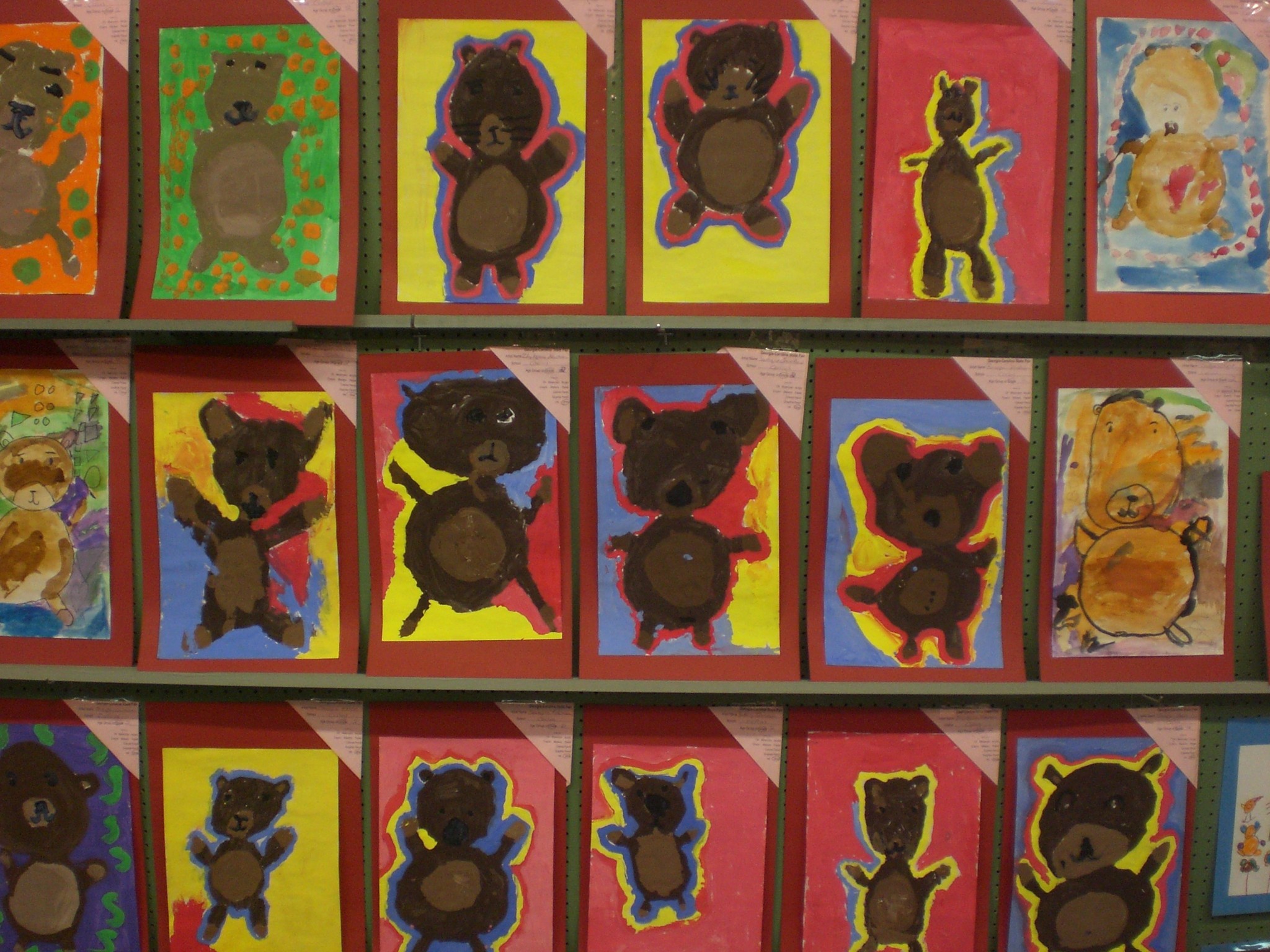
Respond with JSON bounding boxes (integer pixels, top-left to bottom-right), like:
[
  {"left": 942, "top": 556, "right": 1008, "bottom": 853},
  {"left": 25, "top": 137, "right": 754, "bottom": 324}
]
[{"left": 0, "top": 317, "right": 1270, "bottom": 694}]
